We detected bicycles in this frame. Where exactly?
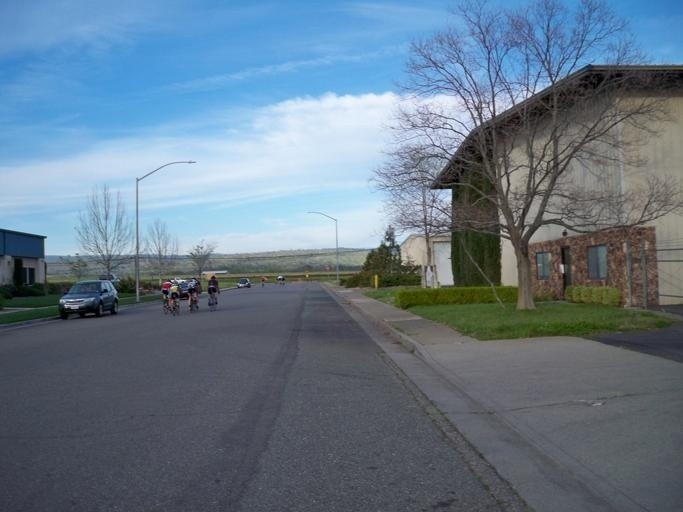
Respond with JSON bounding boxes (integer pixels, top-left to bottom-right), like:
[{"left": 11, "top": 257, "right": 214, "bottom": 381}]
[{"left": 163, "top": 293, "right": 218, "bottom": 316}]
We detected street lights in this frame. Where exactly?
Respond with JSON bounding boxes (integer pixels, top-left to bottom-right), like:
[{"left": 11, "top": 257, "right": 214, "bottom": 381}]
[
  {"left": 134, "top": 159, "right": 197, "bottom": 303},
  {"left": 305, "top": 210, "right": 341, "bottom": 287}
]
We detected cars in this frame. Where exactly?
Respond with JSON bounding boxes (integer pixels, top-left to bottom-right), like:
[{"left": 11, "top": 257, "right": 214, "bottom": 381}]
[
  {"left": 171, "top": 278, "right": 203, "bottom": 300},
  {"left": 58, "top": 279, "right": 120, "bottom": 320},
  {"left": 237, "top": 278, "right": 251, "bottom": 288}
]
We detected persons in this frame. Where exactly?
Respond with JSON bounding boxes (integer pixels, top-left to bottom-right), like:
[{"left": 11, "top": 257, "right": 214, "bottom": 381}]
[{"left": 161, "top": 275, "right": 220, "bottom": 310}]
